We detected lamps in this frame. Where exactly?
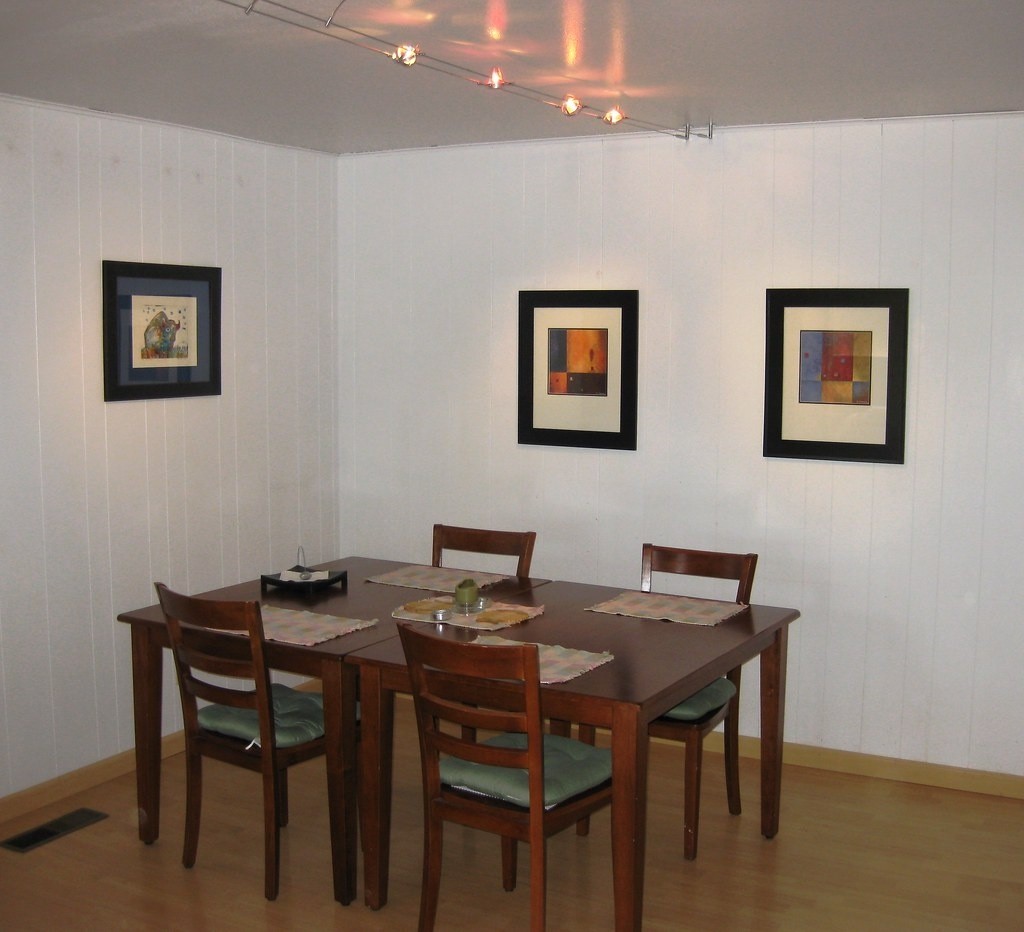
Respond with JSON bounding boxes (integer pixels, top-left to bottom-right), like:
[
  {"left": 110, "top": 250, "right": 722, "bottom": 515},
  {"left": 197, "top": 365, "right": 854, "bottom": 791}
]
[{"left": 392, "top": 45, "right": 629, "bottom": 126}]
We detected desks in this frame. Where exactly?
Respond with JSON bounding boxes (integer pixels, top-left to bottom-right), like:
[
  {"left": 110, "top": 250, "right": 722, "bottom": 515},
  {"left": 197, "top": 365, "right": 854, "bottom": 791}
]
[
  {"left": 117, "top": 556, "right": 551, "bottom": 907},
  {"left": 344, "top": 580, "right": 802, "bottom": 932}
]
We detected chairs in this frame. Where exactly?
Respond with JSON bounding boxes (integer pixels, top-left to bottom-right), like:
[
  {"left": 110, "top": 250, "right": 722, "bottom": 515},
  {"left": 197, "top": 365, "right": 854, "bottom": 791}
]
[
  {"left": 432, "top": 524, "right": 536, "bottom": 745},
  {"left": 154, "top": 582, "right": 362, "bottom": 902},
  {"left": 575, "top": 543, "right": 758, "bottom": 861},
  {"left": 395, "top": 621, "right": 613, "bottom": 932}
]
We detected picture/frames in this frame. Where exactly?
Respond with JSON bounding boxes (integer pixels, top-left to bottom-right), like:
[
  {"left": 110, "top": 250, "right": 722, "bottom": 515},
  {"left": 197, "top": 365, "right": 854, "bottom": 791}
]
[
  {"left": 517, "top": 290, "right": 639, "bottom": 451},
  {"left": 763, "top": 288, "right": 911, "bottom": 465},
  {"left": 102, "top": 260, "right": 222, "bottom": 402}
]
